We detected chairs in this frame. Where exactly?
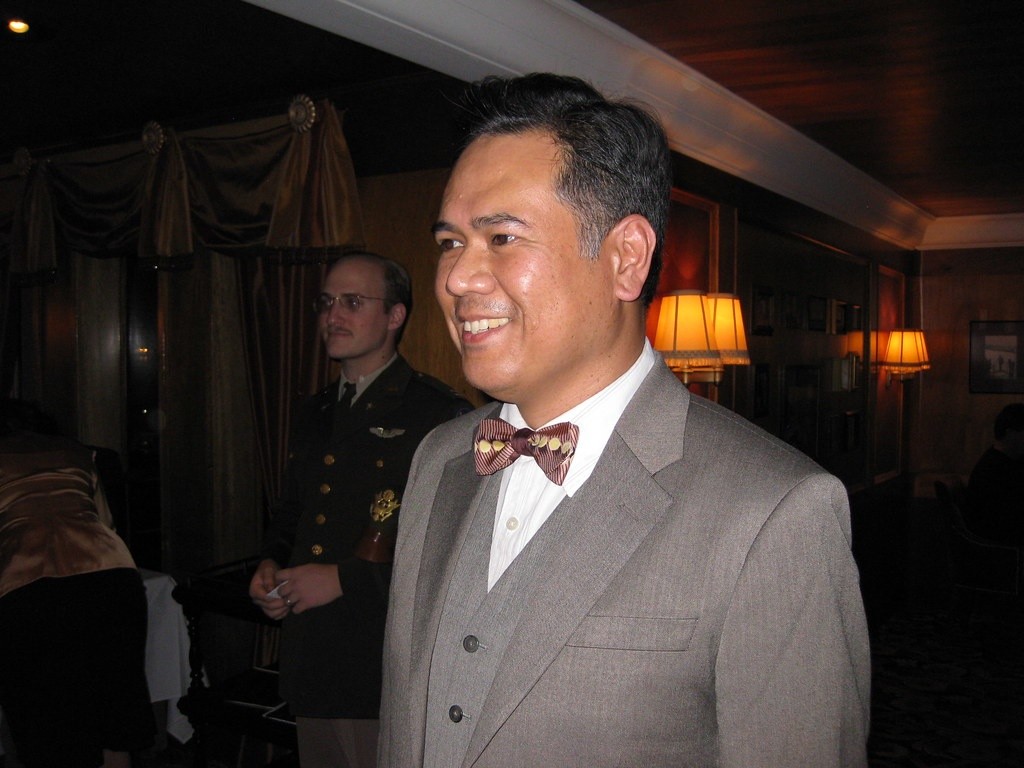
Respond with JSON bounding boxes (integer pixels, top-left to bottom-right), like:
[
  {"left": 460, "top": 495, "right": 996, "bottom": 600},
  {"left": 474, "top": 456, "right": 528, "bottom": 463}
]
[{"left": 932, "top": 478, "right": 1024, "bottom": 617}]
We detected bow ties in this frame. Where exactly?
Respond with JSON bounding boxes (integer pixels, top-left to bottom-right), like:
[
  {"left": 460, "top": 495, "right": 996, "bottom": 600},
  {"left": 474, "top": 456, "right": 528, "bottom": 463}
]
[
  {"left": 336, "top": 382, "right": 357, "bottom": 413},
  {"left": 474, "top": 418, "right": 579, "bottom": 485}
]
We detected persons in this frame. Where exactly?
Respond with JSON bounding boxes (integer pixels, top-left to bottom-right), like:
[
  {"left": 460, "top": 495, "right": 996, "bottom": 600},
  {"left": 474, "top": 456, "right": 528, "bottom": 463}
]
[
  {"left": 248, "top": 249, "right": 476, "bottom": 767},
  {"left": 969, "top": 404, "right": 1024, "bottom": 691},
  {"left": 376, "top": 71, "right": 871, "bottom": 767},
  {"left": 1, "top": 398, "right": 152, "bottom": 768}
]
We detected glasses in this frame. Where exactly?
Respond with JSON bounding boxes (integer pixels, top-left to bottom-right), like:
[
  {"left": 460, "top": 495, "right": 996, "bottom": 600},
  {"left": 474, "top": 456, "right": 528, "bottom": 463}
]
[{"left": 312, "top": 293, "right": 399, "bottom": 313}]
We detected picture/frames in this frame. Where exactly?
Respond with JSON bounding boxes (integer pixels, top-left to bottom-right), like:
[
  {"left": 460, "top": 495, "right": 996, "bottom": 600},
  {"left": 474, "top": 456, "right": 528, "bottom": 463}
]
[{"left": 968, "top": 319, "right": 1024, "bottom": 395}]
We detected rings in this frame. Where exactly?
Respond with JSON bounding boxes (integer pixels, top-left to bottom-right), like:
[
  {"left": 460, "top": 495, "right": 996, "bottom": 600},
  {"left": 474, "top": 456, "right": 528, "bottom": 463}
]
[{"left": 282, "top": 595, "right": 293, "bottom": 607}]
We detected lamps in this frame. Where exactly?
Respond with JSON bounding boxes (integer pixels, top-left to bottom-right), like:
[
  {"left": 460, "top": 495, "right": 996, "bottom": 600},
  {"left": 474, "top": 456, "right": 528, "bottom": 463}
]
[
  {"left": 880, "top": 326, "right": 932, "bottom": 484},
  {"left": 653, "top": 288, "right": 749, "bottom": 412}
]
